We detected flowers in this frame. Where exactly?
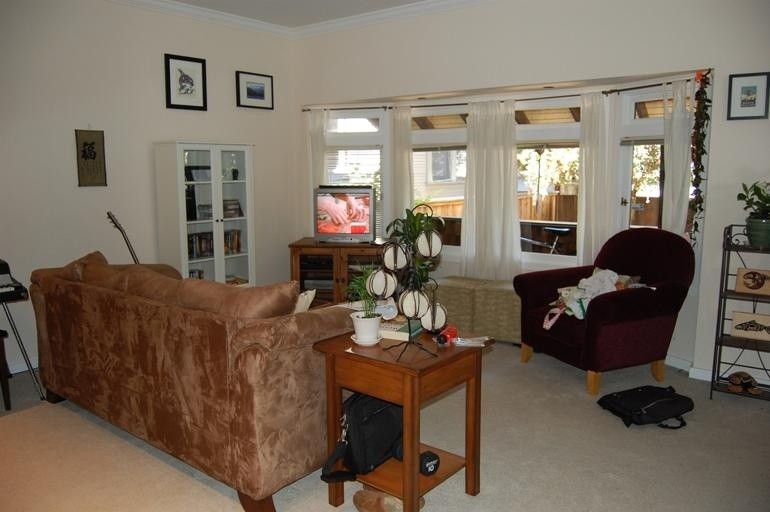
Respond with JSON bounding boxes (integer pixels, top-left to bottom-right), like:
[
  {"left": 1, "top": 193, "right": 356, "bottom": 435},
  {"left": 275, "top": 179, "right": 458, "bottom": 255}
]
[{"left": 681, "top": 72, "right": 713, "bottom": 247}]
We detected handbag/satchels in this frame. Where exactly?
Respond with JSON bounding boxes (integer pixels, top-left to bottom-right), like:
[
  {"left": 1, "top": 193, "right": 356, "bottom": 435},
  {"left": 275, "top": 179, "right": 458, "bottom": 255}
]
[
  {"left": 596, "top": 385, "right": 696, "bottom": 431},
  {"left": 320, "top": 390, "right": 405, "bottom": 484}
]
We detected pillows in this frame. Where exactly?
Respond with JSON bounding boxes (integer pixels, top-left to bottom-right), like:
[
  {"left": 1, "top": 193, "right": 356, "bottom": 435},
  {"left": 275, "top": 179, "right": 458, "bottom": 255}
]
[{"left": 294, "top": 286, "right": 317, "bottom": 312}]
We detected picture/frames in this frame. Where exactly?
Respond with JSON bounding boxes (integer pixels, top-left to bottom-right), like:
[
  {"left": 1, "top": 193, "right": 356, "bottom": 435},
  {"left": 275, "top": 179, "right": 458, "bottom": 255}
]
[
  {"left": 726, "top": 70, "right": 768, "bottom": 124},
  {"left": 162, "top": 50, "right": 210, "bottom": 113},
  {"left": 234, "top": 69, "right": 276, "bottom": 112}
]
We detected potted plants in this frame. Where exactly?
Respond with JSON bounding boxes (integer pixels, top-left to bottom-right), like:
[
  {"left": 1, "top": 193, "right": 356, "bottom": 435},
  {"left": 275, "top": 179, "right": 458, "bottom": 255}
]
[
  {"left": 347, "top": 263, "right": 388, "bottom": 349},
  {"left": 732, "top": 181, "right": 770, "bottom": 246}
]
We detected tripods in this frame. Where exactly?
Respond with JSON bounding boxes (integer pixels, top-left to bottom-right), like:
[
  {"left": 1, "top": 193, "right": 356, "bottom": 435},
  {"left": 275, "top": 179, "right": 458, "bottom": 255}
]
[{"left": 313, "top": 184, "right": 375, "bottom": 244}]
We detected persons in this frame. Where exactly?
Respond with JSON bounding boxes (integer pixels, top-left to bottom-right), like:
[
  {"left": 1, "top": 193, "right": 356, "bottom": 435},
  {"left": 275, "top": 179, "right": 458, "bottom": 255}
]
[{"left": 317, "top": 193, "right": 367, "bottom": 226}]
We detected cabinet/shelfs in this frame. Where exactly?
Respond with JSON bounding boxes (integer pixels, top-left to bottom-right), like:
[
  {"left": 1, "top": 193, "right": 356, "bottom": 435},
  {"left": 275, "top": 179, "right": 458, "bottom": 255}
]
[
  {"left": 706, "top": 221, "right": 770, "bottom": 403},
  {"left": 151, "top": 137, "right": 259, "bottom": 289},
  {"left": 287, "top": 235, "right": 393, "bottom": 307}
]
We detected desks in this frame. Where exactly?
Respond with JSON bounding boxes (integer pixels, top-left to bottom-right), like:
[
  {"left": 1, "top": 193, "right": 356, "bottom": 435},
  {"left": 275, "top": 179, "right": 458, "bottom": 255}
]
[{"left": 306, "top": 326, "right": 499, "bottom": 511}]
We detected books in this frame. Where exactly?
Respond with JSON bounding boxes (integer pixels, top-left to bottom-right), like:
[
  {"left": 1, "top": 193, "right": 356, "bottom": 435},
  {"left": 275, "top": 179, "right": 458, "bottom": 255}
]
[
  {"left": 223, "top": 200, "right": 240, "bottom": 218},
  {"left": 187, "top": 228, "right": 242, "bottom": 260},
  {"left": 378, "top": 317, "right": 424, "bottom": 342}
]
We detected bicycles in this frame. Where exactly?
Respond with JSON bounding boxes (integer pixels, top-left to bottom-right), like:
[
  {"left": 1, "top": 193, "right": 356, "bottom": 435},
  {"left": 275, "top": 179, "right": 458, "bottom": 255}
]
[{"left": 520, "top": 227, "right": 575, "bottom": 255}]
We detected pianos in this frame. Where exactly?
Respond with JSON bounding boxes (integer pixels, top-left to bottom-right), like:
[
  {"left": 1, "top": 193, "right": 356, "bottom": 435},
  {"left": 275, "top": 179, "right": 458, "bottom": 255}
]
[{"left": 0, "top": 260, "right": 29, "bottom": 303}]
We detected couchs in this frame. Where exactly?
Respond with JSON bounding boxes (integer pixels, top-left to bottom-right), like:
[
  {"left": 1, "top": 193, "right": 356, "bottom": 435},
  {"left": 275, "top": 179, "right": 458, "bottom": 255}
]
[{"left": 26, "top": 253, "right": 399, "bottom": 510}]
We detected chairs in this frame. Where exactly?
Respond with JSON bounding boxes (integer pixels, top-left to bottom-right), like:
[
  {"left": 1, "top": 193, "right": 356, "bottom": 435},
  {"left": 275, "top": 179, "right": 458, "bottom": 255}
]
[{"left": 508, "top": 227, "right": 697, "bottom": 394}]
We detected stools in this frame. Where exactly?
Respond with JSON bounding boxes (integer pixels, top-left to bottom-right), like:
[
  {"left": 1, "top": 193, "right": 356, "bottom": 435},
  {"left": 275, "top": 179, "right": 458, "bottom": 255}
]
[
  {"left": 0, "top": 327, "right": 15, "bottom": 413},
  {"left": 469, "top": 276, "right": 523, "bottom": 350},
  {"left": 420, "top": 275, "right": 484, "bottom": 341}
]
